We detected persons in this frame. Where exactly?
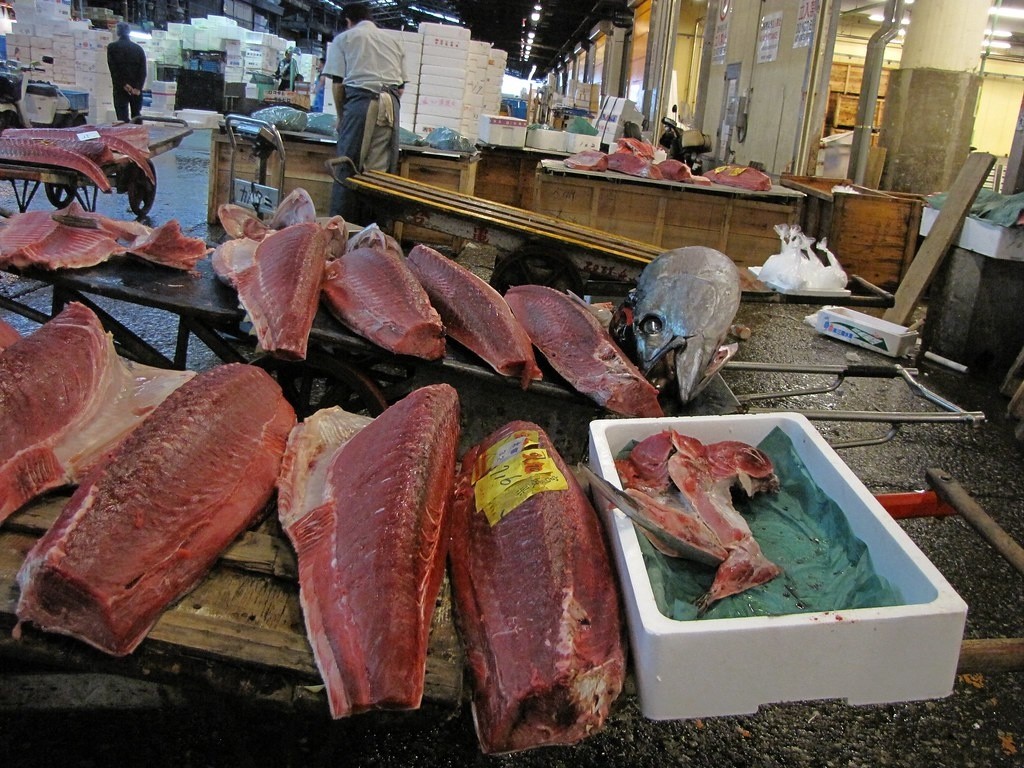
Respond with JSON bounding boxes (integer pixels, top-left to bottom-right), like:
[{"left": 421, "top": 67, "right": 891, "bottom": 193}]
[
  {"left": 323, "top": 2, "right": 411, "bottom": 227},
  {"left": 273, "top": 50, "right": 299, "bottom": 92},
  {"left": 313, "top": 58, "right": 326, "bottom": 112},
  {"left": 107, "top": 23, "right": 147, "bottom": 125}
]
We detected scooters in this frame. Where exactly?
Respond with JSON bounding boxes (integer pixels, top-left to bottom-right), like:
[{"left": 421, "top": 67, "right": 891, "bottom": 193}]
[
  {"left": 659, "top": 104, "right": 713, "bottom": 176},
  {"left": 0, "top": 46, "right": 91, "bottom": 129}
]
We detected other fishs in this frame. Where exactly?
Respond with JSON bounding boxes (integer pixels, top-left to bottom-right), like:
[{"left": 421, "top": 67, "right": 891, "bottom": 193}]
[{"left": 611, "top": 246, "right": 742, "bottom": 404}]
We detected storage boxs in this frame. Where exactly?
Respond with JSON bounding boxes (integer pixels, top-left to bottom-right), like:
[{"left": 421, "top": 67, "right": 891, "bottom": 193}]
[
  {"left": 3, "top": 0, "right": 316, "bottom": 127},
  {"left": 918, "top": 205, "right": 1023, "bottom": 262},
  {"left": 320, "top": 20, "right": 666, "bottom": 165},
  {"left": 812, "top": 305, "right": 917, "bottom": 358},
  {"left": 586, "top": 408, "right": 969, "bottom": 719}
]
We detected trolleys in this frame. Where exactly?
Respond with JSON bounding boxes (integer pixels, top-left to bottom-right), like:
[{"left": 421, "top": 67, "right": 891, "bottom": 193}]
[
  {"left": 0, "top": 115, "right": 193, "bottom": 221},
  {"left": 321, "top": 151, "right": 899, "bottom": 332},
  {"left": 0, "top": 464, "right": 1024, "bottom": 768},
  {"left": 1, "top": 202, "right": 983, "bottom": 431},
  {"left": 215, "top": 114, "right": 287, "bottom": 246}
]
